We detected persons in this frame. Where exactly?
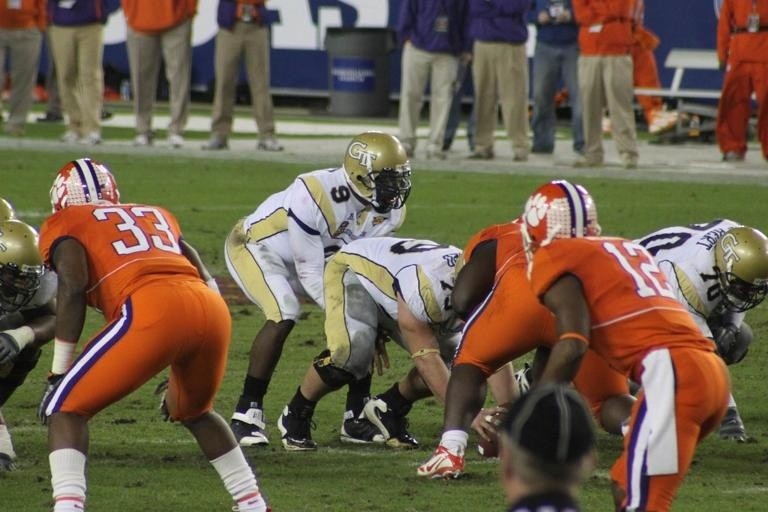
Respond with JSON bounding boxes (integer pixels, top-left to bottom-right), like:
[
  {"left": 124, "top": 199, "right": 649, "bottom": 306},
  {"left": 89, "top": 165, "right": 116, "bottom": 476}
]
[
  {"left": 47, "top": 1, "right": 119, "bottom": 146},
  {"left": 39, "top": 158, "right": 272, "bottom": 512},
  {"left": 122, "top": 0, "right": 198, "bottom": 148},
  {"left": 40, "top": 41, "right": 112, "bottom": 121},
  {"left": 716, "top": 0, "right": 768, "bottom": 167},
  {"left": 209, "top": 0, "right": 284, "bottom": 151},
  {"left": 223, "top": 131, "right": 409, "bottom": 448},
  {"left": 2, "top": 0, "right": 46, "bottom": 134},
  {"left": 1, "top": 222, "right": 62, "bottom": 474}
]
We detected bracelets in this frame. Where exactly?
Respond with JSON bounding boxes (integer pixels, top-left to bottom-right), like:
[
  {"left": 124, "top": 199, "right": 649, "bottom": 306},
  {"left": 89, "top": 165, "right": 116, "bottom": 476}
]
[
  {"left": 49, "top": 337, "right": 77, "bottom": 375},
  {"left": 5, "top": 326, "right": 36, "bottom": 353}
]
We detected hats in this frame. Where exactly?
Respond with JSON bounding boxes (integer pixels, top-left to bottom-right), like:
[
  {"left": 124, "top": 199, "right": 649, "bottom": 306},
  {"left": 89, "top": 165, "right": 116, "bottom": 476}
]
[{"left": 497, "top": 377, "right": 598, "bottom": 465}]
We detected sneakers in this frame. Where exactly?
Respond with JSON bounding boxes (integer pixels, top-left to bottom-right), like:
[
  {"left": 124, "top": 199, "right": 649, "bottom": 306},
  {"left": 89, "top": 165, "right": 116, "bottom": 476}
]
[
  {"left": 224, "top": 394, "right": 273, "bottom": 447},
  {"left": 2, "top": 109, "right": 287, "bottom": 152},
  {"left": 361, "top": 393, "right": 423, "bottom": 449},
  {"left": 395, "top": 108, "right": 694, "bottom": 173},
  {"left": 413, "top": 444, "right": 469, "bottom": 482},
  {"left": 273, "top": 402, "right": 320, "bottom": 452},
  {"left": 336, "top": 407, "right": 391, "bottom": 445}
]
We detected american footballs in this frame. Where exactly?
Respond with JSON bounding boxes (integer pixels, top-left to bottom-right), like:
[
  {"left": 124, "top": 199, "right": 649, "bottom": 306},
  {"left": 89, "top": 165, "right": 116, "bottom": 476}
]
[{"left": 476, "top": 401, "right": 514, "bottom": 456}]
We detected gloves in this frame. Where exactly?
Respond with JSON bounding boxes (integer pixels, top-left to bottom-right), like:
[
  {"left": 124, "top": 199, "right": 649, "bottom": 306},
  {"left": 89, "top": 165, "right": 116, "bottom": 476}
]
[{"left": 0, "top": 325, "right": 36, "bottom": 367}]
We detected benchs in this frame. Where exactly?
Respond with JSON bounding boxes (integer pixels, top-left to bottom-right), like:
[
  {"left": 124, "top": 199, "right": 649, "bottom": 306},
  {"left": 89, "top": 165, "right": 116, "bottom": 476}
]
[{"left": 633, "top": 47, "right": 756, "bottom": 140}]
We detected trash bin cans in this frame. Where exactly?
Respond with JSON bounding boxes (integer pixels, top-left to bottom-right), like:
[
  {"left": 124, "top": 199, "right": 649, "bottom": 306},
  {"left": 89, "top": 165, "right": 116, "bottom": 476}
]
[{"left": 325, "top": 27, "right": 397, "bottom": 116}]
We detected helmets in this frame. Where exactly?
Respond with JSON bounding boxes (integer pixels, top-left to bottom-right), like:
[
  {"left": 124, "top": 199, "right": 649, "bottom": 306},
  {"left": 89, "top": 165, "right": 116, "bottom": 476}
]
[
  {"left": 520, "top": 179, "right": 603, "bottom": 263},
  {"left": 708, "top": 220, "right": 767, "bottom": 314},
  {"left": 342, "top": 130, "right": 416, "bottom": 213},
  {"left": 0, "top": 219, "right": 48, "bottom": 317},
  {"left": 47, "top": 157, "right": 121, "bottom": 215}
]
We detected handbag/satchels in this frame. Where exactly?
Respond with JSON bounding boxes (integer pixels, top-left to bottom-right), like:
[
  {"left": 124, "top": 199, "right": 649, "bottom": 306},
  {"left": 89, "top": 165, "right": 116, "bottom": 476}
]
[{"left": 37, "top": 378, "right": 69, "bottom": 429}]
